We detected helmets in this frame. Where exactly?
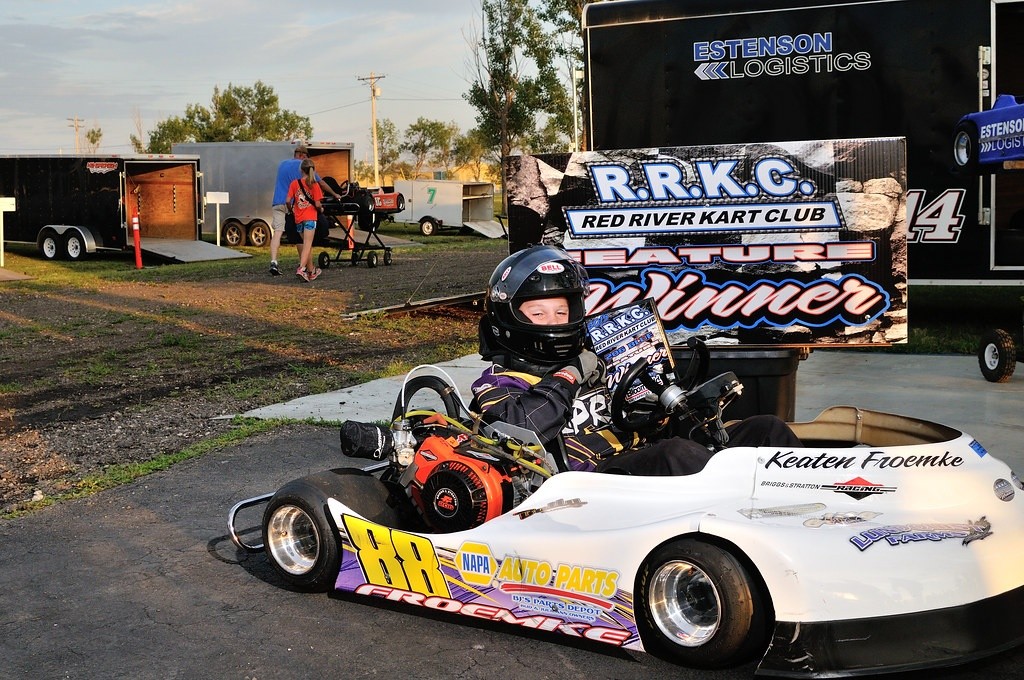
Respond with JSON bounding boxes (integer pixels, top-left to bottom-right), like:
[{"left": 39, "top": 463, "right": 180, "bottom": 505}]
[{"left": 483, "top": 245, "right": 590, "bottom": 365}]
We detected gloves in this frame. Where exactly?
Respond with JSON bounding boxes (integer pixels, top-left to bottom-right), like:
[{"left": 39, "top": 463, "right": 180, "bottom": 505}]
[{"left": 565, "top": 350, "right": 607, "bottom": 388}]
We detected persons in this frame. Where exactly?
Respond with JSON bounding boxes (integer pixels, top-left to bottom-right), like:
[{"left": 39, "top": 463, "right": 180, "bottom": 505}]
[
  {"left": 270, "top": 145, "right": 343, "bottom": 277},
  {"left": 466, "top": 244, "right": 800, "bottom": 477},
  {"left": 286, "top": 159, "right": 322, "bottom": 281}
]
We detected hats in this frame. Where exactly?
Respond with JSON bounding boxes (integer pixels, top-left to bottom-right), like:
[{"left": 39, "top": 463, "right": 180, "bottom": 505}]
[{"left": 295, "top": 146, "right": 310, "bottom": 158}]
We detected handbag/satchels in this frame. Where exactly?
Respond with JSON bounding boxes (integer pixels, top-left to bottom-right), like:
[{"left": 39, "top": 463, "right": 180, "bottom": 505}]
[{"left": 318, "top": 213, "right": 329, "bottom": 239}]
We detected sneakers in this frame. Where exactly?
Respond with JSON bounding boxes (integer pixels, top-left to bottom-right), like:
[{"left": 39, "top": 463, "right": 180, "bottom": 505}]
[
  {"left": 297, "top": 264, "right": 308, "bottom": 274},
  {"left": 308, "top": 267, "right": 322, "bottom": 281},
  {"left": 296, "top": 267, "right": 310, "bottom": 283},
  {"left": 269, "top": 262, "right": 283, "bottom": 275}
]
